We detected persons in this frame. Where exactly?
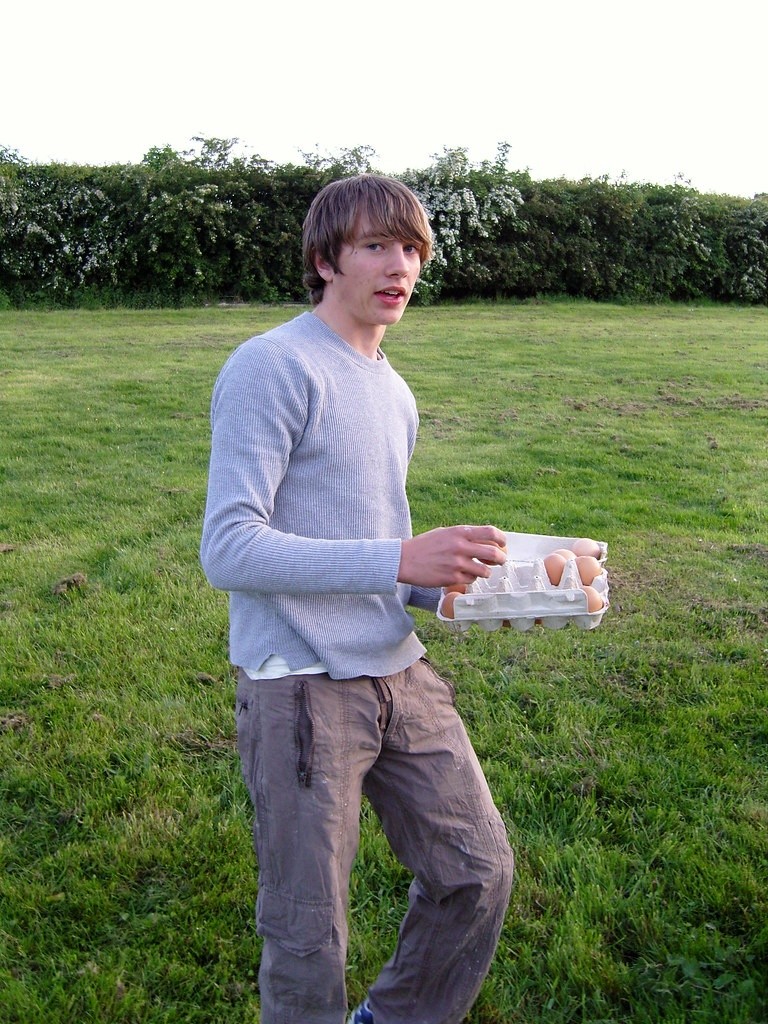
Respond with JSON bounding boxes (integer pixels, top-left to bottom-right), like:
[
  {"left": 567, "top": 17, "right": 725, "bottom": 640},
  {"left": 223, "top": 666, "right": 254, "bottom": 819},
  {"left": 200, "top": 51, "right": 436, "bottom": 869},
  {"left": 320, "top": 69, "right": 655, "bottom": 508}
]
[{"left": 199, "top": 174, "right": 514, "bottom": 1024}]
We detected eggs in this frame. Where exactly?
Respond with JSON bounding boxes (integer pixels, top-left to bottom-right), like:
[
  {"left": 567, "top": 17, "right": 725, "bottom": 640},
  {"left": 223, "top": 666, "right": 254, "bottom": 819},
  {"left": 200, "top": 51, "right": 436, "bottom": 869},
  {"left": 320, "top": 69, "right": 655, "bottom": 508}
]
[
  {"left": 473, "top": 539, "right": 508, "bottom": 566},
  {"left": 543, "top": 538, "right": 603, "bottom": 613},
  {"left": 441, "top": 583, "right": 467, "bottom": 619}
]
